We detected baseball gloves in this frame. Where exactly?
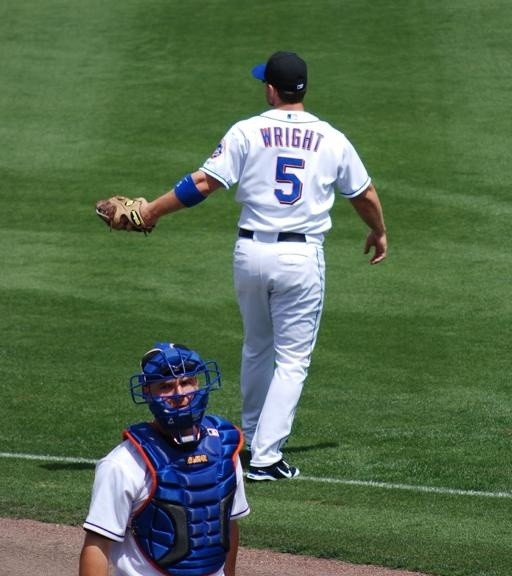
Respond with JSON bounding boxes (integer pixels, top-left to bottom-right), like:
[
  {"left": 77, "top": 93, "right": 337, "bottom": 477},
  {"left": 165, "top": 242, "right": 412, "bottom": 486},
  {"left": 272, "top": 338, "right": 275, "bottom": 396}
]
[{"left": 95, "top": 196, "right": 154, "bottom": 232}]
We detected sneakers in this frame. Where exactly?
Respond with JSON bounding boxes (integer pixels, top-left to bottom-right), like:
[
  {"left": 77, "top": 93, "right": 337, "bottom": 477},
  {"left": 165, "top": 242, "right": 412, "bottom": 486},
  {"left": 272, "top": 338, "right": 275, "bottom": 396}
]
[{"left": 245, "top": 438, "right": 300, "bottom": 481}]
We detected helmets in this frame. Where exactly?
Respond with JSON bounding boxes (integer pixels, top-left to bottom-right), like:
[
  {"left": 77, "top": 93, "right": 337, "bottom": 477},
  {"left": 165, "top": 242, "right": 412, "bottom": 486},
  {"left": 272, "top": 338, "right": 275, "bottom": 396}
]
[{"left": 129, "top": 341, "right": 222, "bottom": 432}]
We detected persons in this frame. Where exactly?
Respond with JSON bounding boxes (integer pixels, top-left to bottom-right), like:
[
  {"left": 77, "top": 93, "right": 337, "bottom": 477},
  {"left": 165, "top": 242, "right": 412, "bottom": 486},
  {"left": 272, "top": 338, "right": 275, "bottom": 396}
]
[
  {"left": 76, "top": 341, "right": 252, "bottom": 576},
  {"left": 94, "top": 50, "right": 388, "bottom": 483}
]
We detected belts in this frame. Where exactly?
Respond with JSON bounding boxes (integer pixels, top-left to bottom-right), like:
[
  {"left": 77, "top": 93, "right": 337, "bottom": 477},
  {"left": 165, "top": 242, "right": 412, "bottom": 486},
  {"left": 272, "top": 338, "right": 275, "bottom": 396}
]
[{"left": 237, "top": 228, "right": 307, "bottom": 242}]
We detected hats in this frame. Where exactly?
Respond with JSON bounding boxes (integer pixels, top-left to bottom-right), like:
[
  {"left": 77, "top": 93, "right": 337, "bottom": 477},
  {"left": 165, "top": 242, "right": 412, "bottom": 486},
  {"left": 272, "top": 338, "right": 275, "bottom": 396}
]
[{"left": 252, "top": 50, "right": 306, "bottom": 91}]
